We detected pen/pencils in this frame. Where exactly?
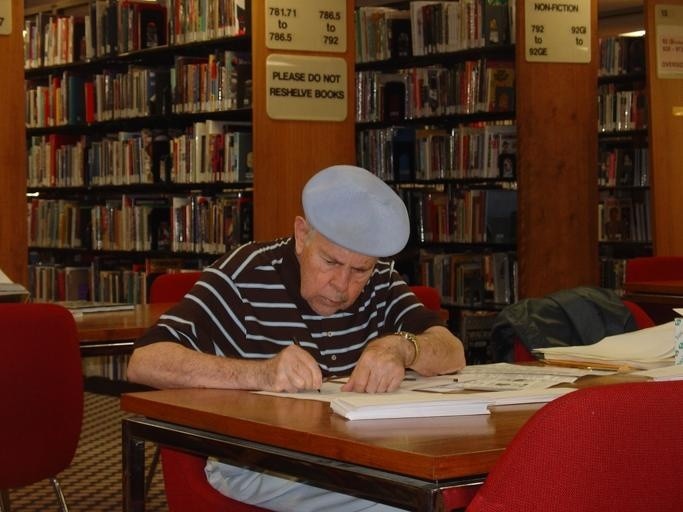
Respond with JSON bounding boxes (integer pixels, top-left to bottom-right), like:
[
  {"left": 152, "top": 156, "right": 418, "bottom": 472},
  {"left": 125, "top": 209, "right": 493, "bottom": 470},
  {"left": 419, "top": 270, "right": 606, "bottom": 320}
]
[{"left": 292, "top": 336, "right": 320, "bottom": 393}]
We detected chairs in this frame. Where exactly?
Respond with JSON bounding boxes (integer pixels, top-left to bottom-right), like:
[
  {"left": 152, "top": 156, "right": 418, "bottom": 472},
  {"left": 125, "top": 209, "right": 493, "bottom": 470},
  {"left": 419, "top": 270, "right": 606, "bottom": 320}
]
[
  {"left": 465, "top": 379, "right": 683, "bottom": 512},
  {"left": 150, "top": 271, "right": 262, "bottom": 512},
  {"left": 1, "top": 302, "right": 87, "bottom": 512}
]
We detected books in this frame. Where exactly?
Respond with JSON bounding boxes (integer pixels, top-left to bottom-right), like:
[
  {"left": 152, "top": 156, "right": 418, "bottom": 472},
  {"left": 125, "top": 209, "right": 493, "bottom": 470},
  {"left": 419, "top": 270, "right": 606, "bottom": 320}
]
[
  {"left": 387, "top": 181, "right": 518, "bottom": 307},
  {"left": 70, "top": 307, "right": 136, "bottom": 384},
  {"left": 22, "top": 120, "right": 252, "bottom": 326},
  {"left": 21, "top": 1, "right": 251, "bottom": 126},
  {"left": 531, "top": 317, "right": 683, "bottom": 366},
  {"left": 458, "top": 308, "right": 498, "bottom": 366},
  {"left": 596, "top": 32, "right": 655, "bottom": 303},
  {"left": 356, "top": 58, "right": 517, "bottom": 177},
  {"left": 354, "top": 0, "right": 516, "bottom": 63}
]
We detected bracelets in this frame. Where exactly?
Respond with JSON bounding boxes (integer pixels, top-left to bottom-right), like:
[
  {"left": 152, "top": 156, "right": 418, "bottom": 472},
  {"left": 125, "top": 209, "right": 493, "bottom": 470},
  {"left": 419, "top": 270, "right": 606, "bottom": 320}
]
[{"left": 390, "top": 324, "right": 422, "bottom": 368}]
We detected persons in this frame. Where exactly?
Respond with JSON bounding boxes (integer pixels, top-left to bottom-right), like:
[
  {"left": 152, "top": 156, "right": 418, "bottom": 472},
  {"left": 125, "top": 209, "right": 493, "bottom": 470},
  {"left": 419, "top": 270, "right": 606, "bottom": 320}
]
[{"left": 121, "top": 157, "right": 469, "bottom": 510}]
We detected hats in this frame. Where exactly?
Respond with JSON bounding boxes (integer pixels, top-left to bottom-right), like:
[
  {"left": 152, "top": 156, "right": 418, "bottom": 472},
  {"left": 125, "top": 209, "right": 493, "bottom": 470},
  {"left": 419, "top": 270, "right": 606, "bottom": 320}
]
[{"left": 302, "top": 161, "right": 412, "bottom": 260}]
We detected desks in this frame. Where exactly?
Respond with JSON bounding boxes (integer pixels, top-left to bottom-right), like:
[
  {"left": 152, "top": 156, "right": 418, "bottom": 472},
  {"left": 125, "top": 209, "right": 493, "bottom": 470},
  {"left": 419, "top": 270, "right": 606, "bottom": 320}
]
[{"left": 118, "top": 360, "right": 652, "bottom": 512}]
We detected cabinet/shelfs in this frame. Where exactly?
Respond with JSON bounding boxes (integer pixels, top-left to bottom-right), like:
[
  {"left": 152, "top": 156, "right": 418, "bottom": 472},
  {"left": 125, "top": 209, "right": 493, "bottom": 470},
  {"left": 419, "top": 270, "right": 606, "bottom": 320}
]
[
  {"left": 357, "top": 3, "right": 594, "bottom": 356},
  {"left": 596, "top": 7, "right": 682, "bottom": 333},
  {"left": 25, "top": 0, "right": 355, "bottom": 397}
]
